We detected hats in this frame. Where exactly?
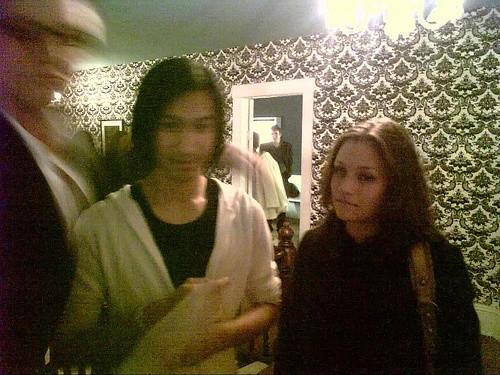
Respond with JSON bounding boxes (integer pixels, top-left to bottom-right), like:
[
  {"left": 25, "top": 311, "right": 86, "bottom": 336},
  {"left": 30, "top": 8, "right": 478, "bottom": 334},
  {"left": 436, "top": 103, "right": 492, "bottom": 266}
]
[{"left": 0, "top": 0, "right": 107, "bottom": 50}]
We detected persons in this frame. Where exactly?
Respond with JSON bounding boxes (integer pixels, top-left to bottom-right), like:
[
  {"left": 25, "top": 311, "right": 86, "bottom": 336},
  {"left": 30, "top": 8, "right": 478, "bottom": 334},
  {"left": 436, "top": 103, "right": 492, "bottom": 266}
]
[
  {"left": 274, "top": 118, "right": 483, "bottom": 375},
  {"left": 0, "top": 0, "right": 293, "bottom": 375}
]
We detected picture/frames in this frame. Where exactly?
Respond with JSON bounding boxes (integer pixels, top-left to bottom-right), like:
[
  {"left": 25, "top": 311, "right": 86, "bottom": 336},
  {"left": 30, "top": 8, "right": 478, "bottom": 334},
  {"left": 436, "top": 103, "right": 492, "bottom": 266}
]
[{"left": 100, "top": 118, "right": 124, "bottom": 153}]
